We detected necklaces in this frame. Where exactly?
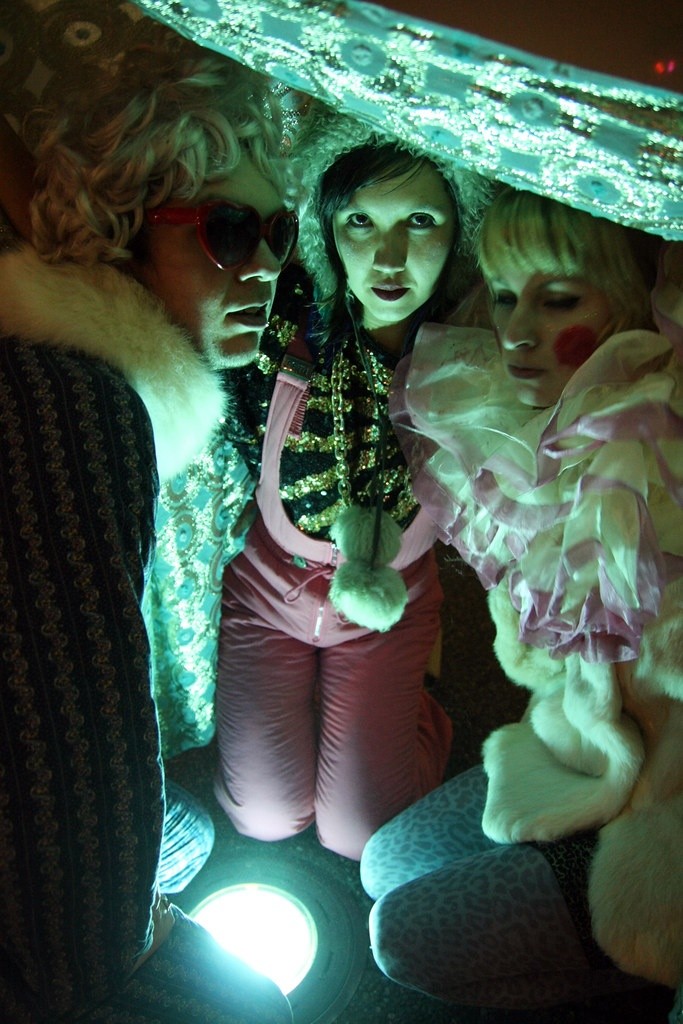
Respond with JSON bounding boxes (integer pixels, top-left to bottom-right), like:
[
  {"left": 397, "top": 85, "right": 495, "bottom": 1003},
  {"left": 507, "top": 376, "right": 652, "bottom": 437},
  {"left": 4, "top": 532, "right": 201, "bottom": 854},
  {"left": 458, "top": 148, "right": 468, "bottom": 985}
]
[{"left": 331, "top": 348, "right": 409, "bottom": 517}]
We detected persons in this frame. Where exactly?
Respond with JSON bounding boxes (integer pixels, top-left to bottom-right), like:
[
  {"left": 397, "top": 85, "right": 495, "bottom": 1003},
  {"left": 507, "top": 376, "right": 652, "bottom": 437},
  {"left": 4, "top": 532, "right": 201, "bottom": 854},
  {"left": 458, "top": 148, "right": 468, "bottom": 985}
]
[
  {"left": 360, "top": 189, "right": 683, "bottom": 1024},
  {"left": 216, "top": 111, "right": 493, "bottom": 858},
  {"left": 0, "top": 46, "right": 301, "bottom": 1024}
]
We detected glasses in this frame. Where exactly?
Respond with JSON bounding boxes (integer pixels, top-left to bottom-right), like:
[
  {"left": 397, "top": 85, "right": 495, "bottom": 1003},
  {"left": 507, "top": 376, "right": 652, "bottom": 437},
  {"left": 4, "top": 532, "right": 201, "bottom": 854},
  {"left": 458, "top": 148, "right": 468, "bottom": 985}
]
[{"left": 148, "top": 197, "right": 299, "bottom": 272}]
